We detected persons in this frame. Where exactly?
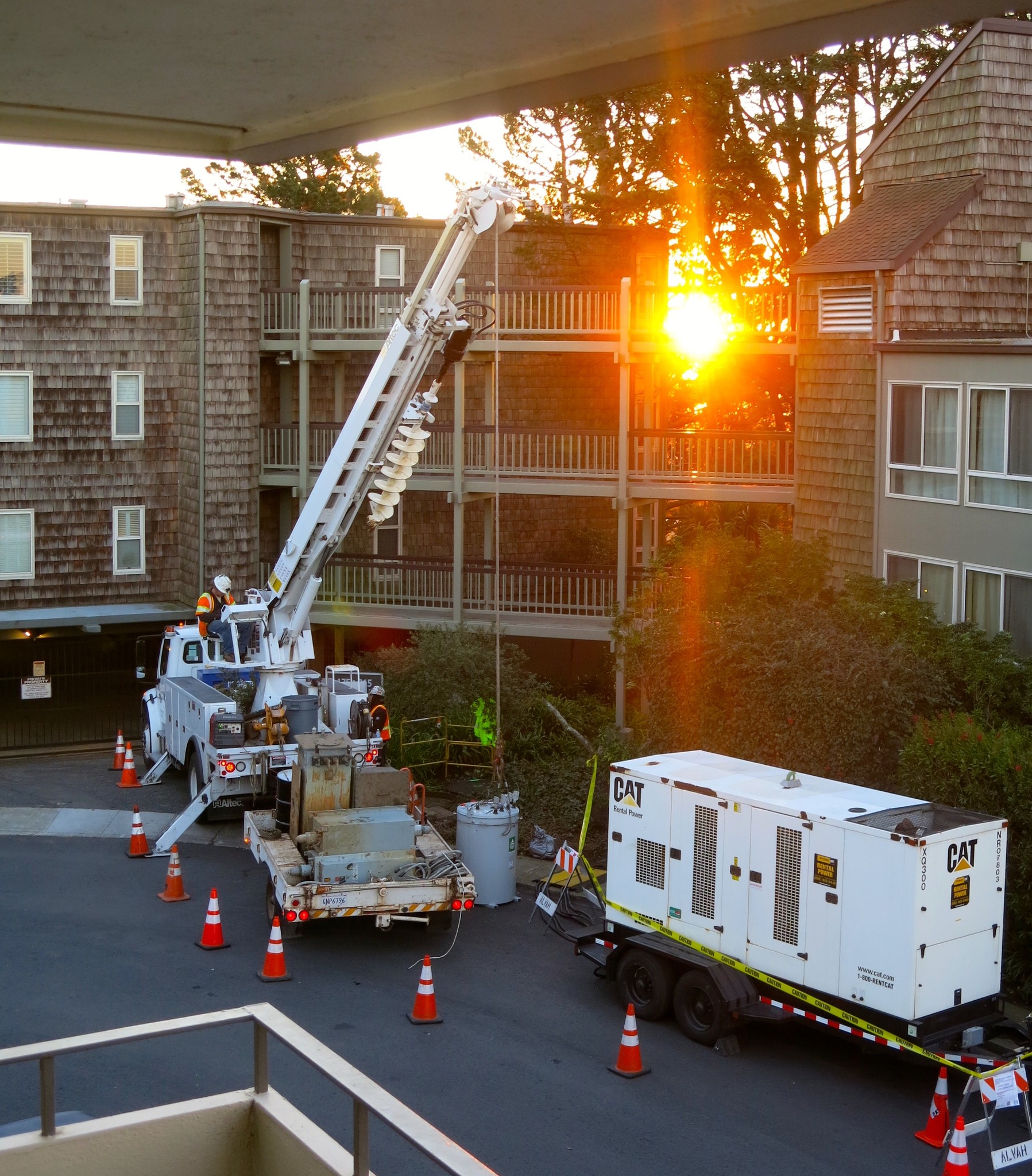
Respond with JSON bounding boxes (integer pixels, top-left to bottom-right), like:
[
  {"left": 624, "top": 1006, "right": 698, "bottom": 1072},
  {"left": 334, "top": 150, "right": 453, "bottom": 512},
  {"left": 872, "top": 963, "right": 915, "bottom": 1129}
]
[
  {"left": 194, "top": 573, "right": 254, "bottom": 664},
  {"left": 369, "top": 685, "right": 391, "bottom": 766}
]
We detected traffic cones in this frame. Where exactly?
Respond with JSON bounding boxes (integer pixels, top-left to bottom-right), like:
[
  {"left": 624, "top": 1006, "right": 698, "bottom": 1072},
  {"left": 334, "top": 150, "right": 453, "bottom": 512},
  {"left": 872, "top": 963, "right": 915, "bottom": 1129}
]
[
  {"left": 256, "top": 916, "right": 294, "bottom": 982},
  {"left": 115, "top": 742, "right": 143, "bottom": 788},
  {"left": 406, "top": 955, "right": 444, "bottom": 1025},
  {"left": 157, "top": 844, "right": 191, "bottom": 902},
  {"left": 943, "top": 1115, "right": 970, "bottom": 1176},
  {"left": 607, "top": 1002, "right": 652, "bottom": 1079},
  {"left": 125, "top": 805, "right": 153, "bottom": 859},
  {"left": 108, "top": 729, "right": 126, "bottom": 770},
  {"left": 194, "top": 887, "right": 231, "bottom": 951},
  {"left": 914, "top": 1066, "right": 949, "bottom": 1150}
]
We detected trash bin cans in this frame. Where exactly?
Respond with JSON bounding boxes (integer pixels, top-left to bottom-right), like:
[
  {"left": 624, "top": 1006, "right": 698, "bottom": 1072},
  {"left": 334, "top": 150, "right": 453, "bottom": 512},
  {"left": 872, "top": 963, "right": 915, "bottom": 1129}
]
[{"left": 281, "top": 694, "right": 319, "bottom": 744}]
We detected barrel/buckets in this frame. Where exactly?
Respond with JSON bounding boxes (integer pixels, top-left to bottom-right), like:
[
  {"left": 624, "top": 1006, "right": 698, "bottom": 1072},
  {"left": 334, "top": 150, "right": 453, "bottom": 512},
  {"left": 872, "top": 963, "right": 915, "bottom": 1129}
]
[
  {"left": 276, "top": 768, "right": 292, "bottom": 835},
  {"left": 281, "top": 694, "right": 320, "bottom": 744}
]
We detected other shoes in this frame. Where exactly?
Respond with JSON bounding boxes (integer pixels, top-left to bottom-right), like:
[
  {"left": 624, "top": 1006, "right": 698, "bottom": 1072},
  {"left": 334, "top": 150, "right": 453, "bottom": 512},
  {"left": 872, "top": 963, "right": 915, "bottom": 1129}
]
[
  {"left": 240, "top": 653, "right": 246, "bottom": 663},
  {"left": 224, "top": 653, "right": 235, "bottom": 662}
]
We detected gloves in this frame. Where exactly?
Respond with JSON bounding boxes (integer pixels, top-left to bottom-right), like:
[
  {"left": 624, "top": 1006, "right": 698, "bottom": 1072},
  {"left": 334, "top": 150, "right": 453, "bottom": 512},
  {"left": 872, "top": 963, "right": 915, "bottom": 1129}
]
[
  {"left": 363, "top": 708, "right": 370, "bottom": 715},
  {"left": 215, "top": 612, "right": 221, "bottom": 620}
]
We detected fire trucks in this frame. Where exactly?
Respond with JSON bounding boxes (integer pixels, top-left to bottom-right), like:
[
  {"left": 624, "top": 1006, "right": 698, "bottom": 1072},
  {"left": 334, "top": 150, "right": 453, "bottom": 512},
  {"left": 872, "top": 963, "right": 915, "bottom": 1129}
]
[{"left": 135, "top": 182, "right": 523, "bottom": 855}]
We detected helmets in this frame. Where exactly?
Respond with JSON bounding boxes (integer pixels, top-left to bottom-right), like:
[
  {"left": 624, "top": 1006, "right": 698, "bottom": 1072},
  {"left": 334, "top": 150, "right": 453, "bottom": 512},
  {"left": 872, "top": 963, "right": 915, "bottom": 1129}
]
[
  {"left": 369, "top": 686, "right": 386, "bottom": 697},
  {"left": 214, "top": 574, "right": 232, "bottom": 593}
]
